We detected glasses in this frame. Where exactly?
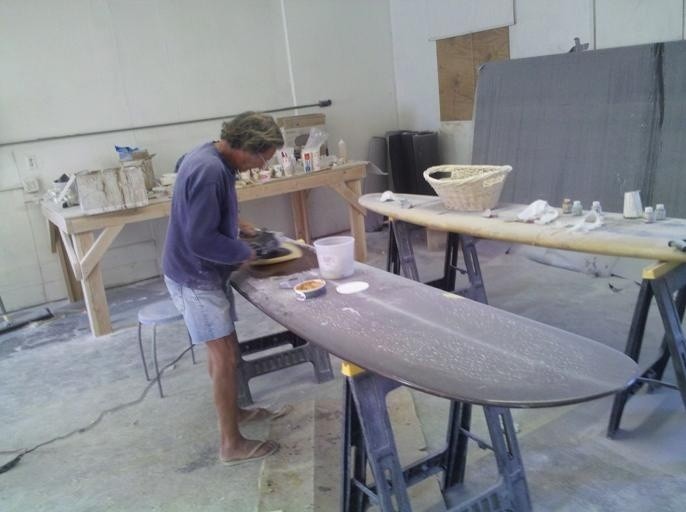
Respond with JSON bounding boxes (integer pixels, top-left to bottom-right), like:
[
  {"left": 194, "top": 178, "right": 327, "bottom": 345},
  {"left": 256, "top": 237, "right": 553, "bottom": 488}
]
[{"left": 255, "top": 151, "right": 269, "bottom": 173}]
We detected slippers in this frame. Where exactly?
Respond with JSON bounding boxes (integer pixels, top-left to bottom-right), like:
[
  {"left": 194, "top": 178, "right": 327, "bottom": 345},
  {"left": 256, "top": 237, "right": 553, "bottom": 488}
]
[
  {"left": 222, "top": 441, "right": 276, "bottom": 467},
  {"left": 236, "top": 407, "right": 269, "bottom": 426}
]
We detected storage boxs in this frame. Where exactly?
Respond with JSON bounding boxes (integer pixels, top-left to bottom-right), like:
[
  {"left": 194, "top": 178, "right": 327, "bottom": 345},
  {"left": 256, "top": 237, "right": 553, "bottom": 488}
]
[{"left": 275, "top": 112, "right": 329, "bottom": 161}]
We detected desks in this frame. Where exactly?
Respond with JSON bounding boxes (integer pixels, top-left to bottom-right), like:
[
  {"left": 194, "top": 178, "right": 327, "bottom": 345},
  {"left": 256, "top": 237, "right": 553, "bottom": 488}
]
[
  {"left": 44, "top": 161, "right": 383, "bottom": 337},
  {"left": 357, "top": 190, "right": 686, "bottom": 437},
  {"left": 221, "top": 247, "right": 637, "bottom": 512}
]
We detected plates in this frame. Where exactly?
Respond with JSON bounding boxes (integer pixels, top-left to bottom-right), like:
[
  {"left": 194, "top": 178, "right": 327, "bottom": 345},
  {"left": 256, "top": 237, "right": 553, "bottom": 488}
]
[
  {"left": 336, "top": 281, "right": 369, "bottom": 295},
  {"left": 294, "top": 278, "right": 326, "bottom": 301}
]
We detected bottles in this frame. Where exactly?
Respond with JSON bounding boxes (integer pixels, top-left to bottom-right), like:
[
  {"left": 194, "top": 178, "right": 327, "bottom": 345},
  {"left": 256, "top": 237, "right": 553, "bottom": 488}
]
[
  {"left": 233, "top": 136, "right": 350, "bottom": 188},
  {"left": 562, "top": 188, "right": 667, "bottom": 223}
]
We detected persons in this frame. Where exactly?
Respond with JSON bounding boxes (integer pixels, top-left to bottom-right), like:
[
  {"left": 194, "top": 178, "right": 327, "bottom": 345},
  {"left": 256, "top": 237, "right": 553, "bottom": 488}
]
[{"left": 162, "top": 111, "right": 292, "bottom": 463}]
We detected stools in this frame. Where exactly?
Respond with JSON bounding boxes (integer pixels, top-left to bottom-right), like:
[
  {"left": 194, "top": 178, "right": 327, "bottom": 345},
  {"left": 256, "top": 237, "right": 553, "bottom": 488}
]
[{"left": 137, "top": 302, "right": 195, "bottom": 397}]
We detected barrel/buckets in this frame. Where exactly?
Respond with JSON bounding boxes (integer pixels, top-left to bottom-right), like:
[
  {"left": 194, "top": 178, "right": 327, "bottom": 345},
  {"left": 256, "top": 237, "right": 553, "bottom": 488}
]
[{"left": 313, "top": 235, "right": 355, "bottom": 278}]
